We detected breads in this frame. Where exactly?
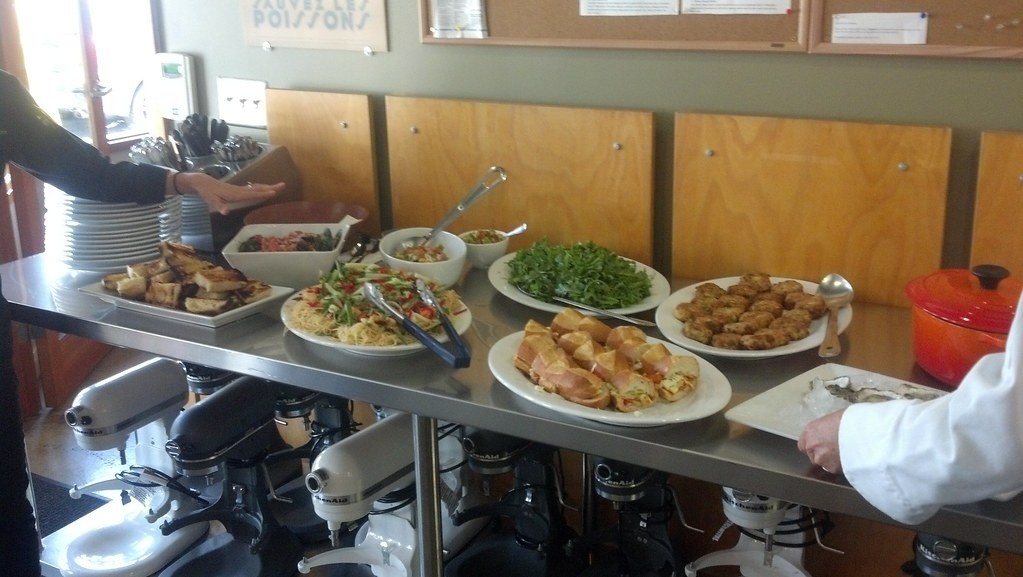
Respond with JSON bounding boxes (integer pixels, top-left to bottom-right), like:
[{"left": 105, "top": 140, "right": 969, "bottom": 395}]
[{"left": 101, "top": 242, "right": 273, "bottom": 316}]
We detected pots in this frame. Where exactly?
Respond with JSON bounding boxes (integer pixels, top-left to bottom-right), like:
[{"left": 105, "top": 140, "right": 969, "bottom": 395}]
[{"left": 905, "top": 265, "right": 1023, "bottom": 387}]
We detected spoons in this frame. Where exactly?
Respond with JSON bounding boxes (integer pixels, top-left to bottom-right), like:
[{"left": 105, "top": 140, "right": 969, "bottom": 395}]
[
  {"left": 815, "top": 273, "right": 853, "bottom": 357},
  {"left": 517, "top": 282, "right": 658, "bottom": 329},
  {"left": 356, "top": 239, "right": 379, "bottom": 263},
  {"left": 347, "top": 243, "right": 365, "bottom": 263}
]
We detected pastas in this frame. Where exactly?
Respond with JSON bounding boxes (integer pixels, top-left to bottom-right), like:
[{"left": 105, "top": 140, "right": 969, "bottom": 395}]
[{"left": 289, "top": 287, "right": 462, "bottom": 347}]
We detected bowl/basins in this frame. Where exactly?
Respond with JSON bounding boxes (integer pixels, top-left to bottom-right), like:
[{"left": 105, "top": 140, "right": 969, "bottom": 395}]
[
  {"left": 222, "top": 223, "right": 350, "bottom": 271},
  {"left": 459, "top": 229, "right": 509, "bottom": 268},
  {"left": 380, "top": 227, "right": 467, "bottom": 287}
]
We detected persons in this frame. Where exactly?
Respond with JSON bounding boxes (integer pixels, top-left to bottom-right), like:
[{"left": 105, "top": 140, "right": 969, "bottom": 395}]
[
  {"left": 0, "top": 69, "right": 285, "bottom": 576},
  {"left": 798, "top": 283, "right": 1023, "bottom": 525}
]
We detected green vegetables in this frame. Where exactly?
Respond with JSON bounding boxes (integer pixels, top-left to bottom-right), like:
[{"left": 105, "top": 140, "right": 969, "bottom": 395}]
[
  {"left": 508, "top": 234, "right": 652, "bottom": 310},
  {"left": 316, "top": 258, "right": 447, "bottom": 344},
  {"left": 238, "top": 227, "right": 341, "bottom": 253}
]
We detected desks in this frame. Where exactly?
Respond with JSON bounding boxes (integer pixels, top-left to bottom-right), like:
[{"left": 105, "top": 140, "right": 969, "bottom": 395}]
[{"left": 0, "top": 244, "right": 1023, "bottom": 577}]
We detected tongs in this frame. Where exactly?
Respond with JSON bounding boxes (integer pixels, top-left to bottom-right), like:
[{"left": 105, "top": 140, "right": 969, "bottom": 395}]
[{"left": 364, "top": 278, "right": 471, "bottom": 369}]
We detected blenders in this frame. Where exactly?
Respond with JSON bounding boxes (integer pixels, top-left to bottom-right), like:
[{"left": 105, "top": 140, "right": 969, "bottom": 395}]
[{"left": 40, "top": 358, "right": 996, "bottom": 577}]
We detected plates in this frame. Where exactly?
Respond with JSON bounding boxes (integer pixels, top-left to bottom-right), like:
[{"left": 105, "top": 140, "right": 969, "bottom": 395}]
[
  {"left": 488, "top": 252, "right": 671, "bottom": 319},
  {"left": 655, "top": 276, "right": 853, "bottom": 361},
  {"left": 280, "top": 285, "right": 471, "bottom": 357},
  {"left": 43, "top": 182, "right": 212, "bottom": 273},
  {"left": 487, "top": 326, "right": 732, "bottom": 428},
  {"left": 78, "top": 282, "right": 295, "bottom": 327},
  {"left": 724, "top": 363, "right": 949, "bottom": 442}
]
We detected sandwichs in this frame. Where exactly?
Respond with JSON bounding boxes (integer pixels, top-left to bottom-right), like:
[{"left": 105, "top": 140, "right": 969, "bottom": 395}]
[{"left": 515, "top": 308, "right": 700, "bottom": 413}]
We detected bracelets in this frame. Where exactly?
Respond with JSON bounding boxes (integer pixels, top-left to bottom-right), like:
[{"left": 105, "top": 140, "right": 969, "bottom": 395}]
[{"left": 174, "top": 172, "right": 185, "bottom": 195}]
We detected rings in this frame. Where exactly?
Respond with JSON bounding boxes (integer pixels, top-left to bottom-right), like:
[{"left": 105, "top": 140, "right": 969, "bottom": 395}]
[
  {"left": 822, "top": 467, "right": 828, "bottom": 471},
  {"left": 248, "top": 182, "right": 253, "bottom": 188}
]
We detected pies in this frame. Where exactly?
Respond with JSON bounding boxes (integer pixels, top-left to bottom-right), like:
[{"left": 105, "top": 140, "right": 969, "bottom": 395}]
[{"left": 674, "top": 272, "right": 826, "bottom": 350}]
